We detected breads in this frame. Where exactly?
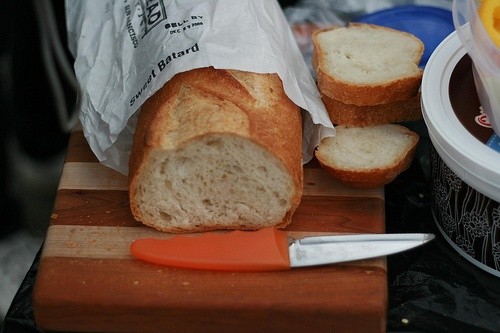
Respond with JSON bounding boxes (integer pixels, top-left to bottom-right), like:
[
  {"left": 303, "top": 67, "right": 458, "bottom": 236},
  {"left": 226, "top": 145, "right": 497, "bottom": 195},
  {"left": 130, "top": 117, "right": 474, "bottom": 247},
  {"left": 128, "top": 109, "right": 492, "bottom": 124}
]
[
  {"left": 312, "top": 21, "right": 425, "bottom": 126},
  {"left": 315, "top": 122, "right": 419, "bottom": 184},
  {"left": 128, "top": 69, "right": 303, "bottom": 234}
]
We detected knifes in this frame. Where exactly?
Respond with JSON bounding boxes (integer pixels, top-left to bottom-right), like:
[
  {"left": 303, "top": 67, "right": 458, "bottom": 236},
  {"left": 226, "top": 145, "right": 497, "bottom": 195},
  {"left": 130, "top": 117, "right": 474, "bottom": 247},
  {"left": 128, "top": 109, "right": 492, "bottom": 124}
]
[{"left": 128, "top": 226, "right": 436, "bottom": 271}]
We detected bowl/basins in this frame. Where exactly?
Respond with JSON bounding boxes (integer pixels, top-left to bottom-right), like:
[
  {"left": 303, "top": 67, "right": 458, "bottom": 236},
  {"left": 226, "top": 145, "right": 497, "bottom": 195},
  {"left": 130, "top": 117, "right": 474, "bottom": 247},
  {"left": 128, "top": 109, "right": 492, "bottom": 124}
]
[{"left": 453, "top": 0, "right": 500, "bottom": 143}]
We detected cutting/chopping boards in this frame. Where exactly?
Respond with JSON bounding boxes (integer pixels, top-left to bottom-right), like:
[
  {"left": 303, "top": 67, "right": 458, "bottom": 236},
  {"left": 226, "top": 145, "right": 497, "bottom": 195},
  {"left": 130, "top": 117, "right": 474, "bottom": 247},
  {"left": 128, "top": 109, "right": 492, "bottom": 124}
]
[{"left": 32, "top": 117, "right": 386, "bottom": 333}]
